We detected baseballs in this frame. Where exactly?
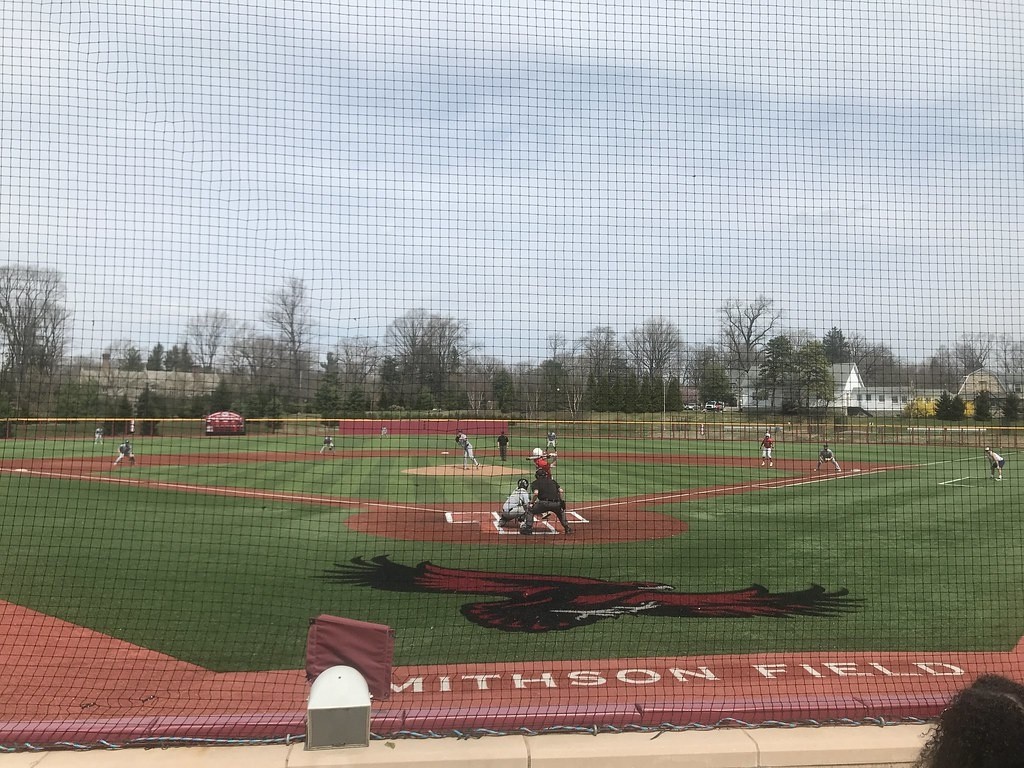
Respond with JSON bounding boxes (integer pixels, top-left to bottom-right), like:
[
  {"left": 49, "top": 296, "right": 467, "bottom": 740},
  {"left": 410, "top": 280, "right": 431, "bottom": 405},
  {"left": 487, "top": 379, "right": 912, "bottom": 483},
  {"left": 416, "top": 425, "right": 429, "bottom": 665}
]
[{"left": 556, "top": 388, "right": 560, "bottom": 392}]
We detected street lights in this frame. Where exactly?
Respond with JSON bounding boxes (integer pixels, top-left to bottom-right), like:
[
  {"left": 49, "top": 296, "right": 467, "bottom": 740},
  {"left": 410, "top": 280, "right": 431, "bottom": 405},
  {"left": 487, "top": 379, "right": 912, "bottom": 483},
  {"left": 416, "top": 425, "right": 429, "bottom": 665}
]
[{"left": 661, "top": 373, "right": 670, "bottom": 432}]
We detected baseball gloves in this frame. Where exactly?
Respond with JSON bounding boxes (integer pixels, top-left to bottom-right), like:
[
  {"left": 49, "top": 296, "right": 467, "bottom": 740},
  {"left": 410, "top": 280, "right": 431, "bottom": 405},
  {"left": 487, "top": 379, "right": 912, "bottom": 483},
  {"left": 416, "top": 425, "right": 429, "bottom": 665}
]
[
  {"left": 455, "top": 435, "right": 462, "bottom": 442},
  {"left": 825, "top": 457, "right": 831, "bottom": 462}
]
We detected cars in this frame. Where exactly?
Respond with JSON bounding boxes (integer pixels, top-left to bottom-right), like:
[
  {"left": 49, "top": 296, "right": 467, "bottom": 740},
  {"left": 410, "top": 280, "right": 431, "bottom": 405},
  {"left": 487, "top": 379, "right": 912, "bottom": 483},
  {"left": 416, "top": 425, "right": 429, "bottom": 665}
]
[
  {"left": 705, "top": 401, "right": 725, "bottom": 410},
  {"left": 684, "top": 403, "right": 699, "bottom": 410}
]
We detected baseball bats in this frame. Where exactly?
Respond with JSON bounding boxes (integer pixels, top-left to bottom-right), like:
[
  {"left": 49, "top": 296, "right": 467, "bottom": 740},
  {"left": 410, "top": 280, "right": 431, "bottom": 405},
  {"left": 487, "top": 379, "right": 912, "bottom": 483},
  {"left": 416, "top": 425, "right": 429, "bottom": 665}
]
[{"left": 526, "top": 452, "right": 558, "bottom": 460}]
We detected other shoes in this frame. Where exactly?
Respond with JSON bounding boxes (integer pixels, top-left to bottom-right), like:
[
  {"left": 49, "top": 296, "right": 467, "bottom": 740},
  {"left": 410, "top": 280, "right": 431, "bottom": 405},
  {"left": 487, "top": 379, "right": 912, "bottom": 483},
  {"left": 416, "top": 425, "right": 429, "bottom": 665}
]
[
  {"left": 542, "top": 512, "right": 551, "bottom": 518},
  {"left": 499, "top": 519, "right": 506, "bottom": 527},
  {"left": 770, "top": 462, "right": 773, "bottom": 467},
  {"left": 762, "top": 462, "right": 765, "bottom": 466},
  {"left": 837, "top": 469, "right": 842, "bottom": 472},
  {"left": 814, "top": 468, "right": 817, "bottom": 472},
  {"left": 501, "top": 457, "right": 506, "bottom": 461},
  {"left": 520, "top": 522, "right": 527, "bottom": 528},
  {"left": 995, "top": 478, "right": 1001, "bottom": 481},
  {"left": 564, "top": 526, "right": 572, "bottom": 533},
  {"left": 521, "top": 526, "right": 532, "bottom": 535}
]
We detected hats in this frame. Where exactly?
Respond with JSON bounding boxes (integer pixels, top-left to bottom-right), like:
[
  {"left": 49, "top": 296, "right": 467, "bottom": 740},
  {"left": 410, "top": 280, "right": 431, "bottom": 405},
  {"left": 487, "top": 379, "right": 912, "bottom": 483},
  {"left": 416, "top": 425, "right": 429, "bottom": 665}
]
[{"left": 985, "top": 446, "right": 989, "bottom": 451}]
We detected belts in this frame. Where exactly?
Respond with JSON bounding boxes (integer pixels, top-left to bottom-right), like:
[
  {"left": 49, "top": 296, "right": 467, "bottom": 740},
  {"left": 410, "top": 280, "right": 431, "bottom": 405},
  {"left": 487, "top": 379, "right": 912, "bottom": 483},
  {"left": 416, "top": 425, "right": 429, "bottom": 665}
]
[{"left": 540, "top": 498, "right": 559, "bottom": 502}]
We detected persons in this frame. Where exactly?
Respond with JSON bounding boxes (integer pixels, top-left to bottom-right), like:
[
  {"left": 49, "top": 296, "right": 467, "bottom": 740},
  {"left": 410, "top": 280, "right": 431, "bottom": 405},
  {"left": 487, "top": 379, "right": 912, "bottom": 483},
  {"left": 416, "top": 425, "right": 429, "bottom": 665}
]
[
  {"left": 912, "top": 674, "right": 1024, "bottom": 768},
  {"left": 764, "top": 429, "right": 771, "bottom": 439},
  {"left": 543, "top": 429, "right": 558, "bottom": 454},
  {"left": 497, "top": 431, "right": 509, "bottom": 461},
  {"left": 93, "top": 425, "right": 104, "bottom": 445},
  {"left": 868, "top": 421, "right": 875, "bottom": 433},
  {"left": 455, "top": 428, "right": 479, "bottom": 470},
  {"left": 113, "top": 440, "right": 136, "bottom": 466},
  {"left": 380, "top": 425, "right": 389, "bottom": 439},
  {"left": 760, "top": 436, "right": 774, "bottom": 467},
  {"left": 520, "top": 469, "right": 573, "bottom": 534},
  {"left": 319, "top": 436, "right": 336, "bottom": 456},
  {"left": 532, "top": 447, "right": 557, "bottom": 479},
  {"left": 814, "top": 445, "right": 842, "bottom": 472},
  {"left": 498, "top": 478, "right": 536, "bottom": 531},
  {"left": 984, "top": 447, "right": 1005, "bottom": 482}
]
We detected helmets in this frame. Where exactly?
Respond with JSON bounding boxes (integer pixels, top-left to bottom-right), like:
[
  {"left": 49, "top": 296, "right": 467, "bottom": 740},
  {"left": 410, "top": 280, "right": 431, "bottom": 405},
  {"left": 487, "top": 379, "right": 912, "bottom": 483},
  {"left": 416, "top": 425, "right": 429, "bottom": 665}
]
[
  {"left": 765, "top": 433, "right": 770, "bottom": 438},
  {"left": 824, "top": 444, "right": 828, "bottom": 450},
  {"left": 535, "top": 470, "right": 547, "bottom": 478},
  {"left": 518, "top": 479, "right": 528, "bottom": 489},
  {"left": 533, "top": 447, "right": 542, "bottom": 456}
]
